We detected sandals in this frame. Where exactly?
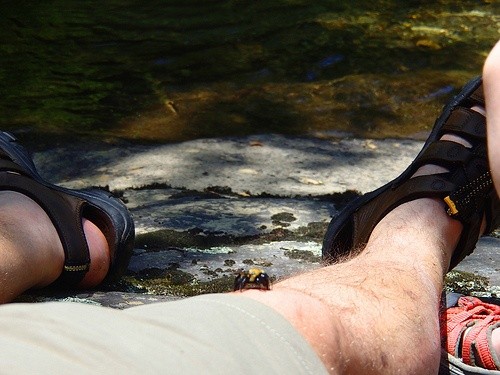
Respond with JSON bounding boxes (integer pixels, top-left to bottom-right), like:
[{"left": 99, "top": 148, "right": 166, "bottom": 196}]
[
  {"left": 322, "top": 75, "right": 500, "bottom": 272},
  {"left": 0, "top": 129, "right": 135, "bottom": 298}
]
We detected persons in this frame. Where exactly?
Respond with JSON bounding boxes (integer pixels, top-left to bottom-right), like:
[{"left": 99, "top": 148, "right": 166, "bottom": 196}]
[{"left": 0, "top": 74, "right": 500, "bottom": 375}]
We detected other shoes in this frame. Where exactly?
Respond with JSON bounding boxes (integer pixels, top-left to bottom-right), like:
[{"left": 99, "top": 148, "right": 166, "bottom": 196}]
[{"left": 438, "top": 290, "right": 500, "bottom": 375}]
